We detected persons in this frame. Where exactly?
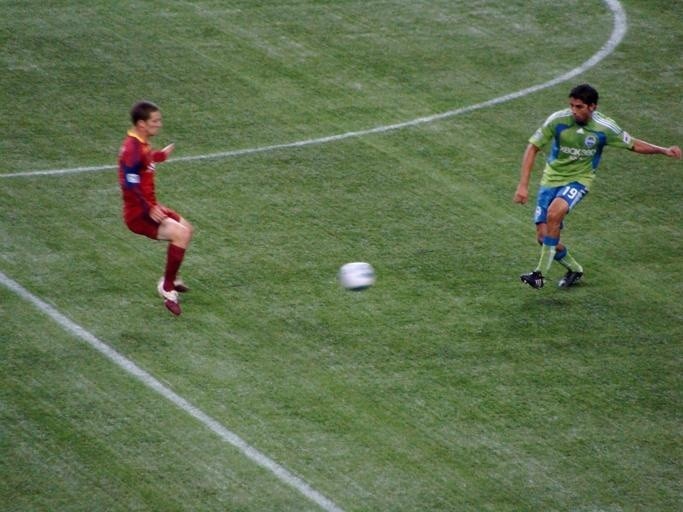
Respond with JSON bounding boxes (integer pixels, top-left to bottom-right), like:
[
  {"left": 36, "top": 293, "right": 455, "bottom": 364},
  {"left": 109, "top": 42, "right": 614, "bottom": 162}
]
[
  {"left": 118, "top": 101, "right": 193, "bottom": 315},
  {"left": 513, "top": 84, "right": 681, "bottom": 290}
]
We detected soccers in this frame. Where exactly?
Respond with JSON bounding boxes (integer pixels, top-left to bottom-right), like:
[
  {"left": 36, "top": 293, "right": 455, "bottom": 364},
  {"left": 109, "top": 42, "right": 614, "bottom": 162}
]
[{"left": 340, "top": 262, "right": 374, "bottom": 291}]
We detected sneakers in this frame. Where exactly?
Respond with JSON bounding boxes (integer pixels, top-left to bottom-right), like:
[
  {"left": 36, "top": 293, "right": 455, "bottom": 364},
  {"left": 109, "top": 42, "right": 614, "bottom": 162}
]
[
  {"left": 157, "top": 282, "right": 181, "bottom": 315},
  {"left": 160, "top": 276, "right": 190, "bottom": 292},
  {"left": 559, "top": 272, "right": 583, "bottom": 288},
  {"left": 520, "top": 271, "right": 544, "bottom": 289}
]
